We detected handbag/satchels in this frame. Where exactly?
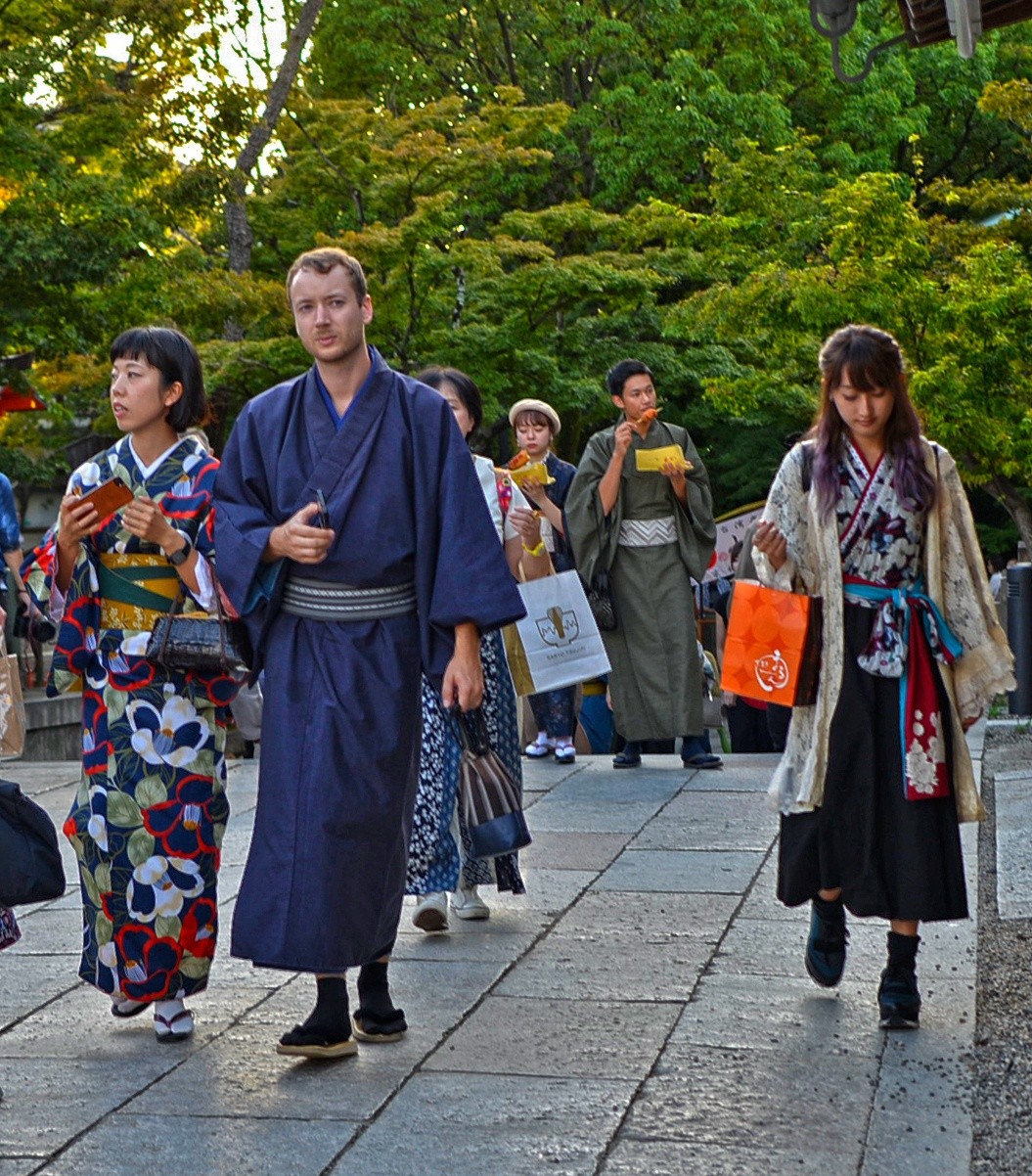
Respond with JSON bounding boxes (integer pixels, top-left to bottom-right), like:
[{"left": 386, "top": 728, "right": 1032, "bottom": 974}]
[
  {"left": 143, "top": 590, "right": 246, "bottom": 670},
  {"left": 719, "top": 571, "right": 820, "bottom": 709},
  {"left": 504, "top": 545, "right": 612, "bottom": 696},
  {"left": 0, "top": 627, "right": 26, "bottom": 761},
  {"left": 455, "top": 703, "right": 532, "bottom": 862}
]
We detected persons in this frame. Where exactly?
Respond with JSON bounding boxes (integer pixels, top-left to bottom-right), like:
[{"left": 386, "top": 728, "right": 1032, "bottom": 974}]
[
  {"left": 508, "top": 399, "right": 578, "bottom": 764},
  {"left": 402, "top": 367, "right": 550, "bottom": 933},
  {"left": 0, "top": 474, "right": 70, "bottom": 695},
  {"left": 751, "top": 323, "right": 1012, "bottom": 1035},
  {"left": 578, "top": 538, "right": 787, "bottom": 755},
  {"left": 23, "top": 325, "right": 239, "bottom": 1041},
  {"left": 566, "top": 358, "right": 724, "bottom": 773},
  {"left": 213, "top": 245, "right": 532, "bottom": 1057}
]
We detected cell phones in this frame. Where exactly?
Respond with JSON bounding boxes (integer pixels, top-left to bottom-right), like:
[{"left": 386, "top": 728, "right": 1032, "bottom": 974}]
[
  {"left": 67, "top": 476, "right": 134, "bottom": 530},
  {"left": 315, "top": 489, "right": 329, "bottom": 529}
]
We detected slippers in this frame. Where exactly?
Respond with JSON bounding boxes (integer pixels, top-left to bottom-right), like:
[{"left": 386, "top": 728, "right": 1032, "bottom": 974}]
[
  {"left": 684, "top": 754, "right": 724, "bottom": 768},
  {"left": 153, "top": 1007, "right": 192, "bottom": 1041},
  {"left": 526, "top": 741, "right": 553, "bottom": 757},
  {"left": 554, "top": 743, "right": 575, "bottom": 762},
  {"left": 351, "top": 1007, "right": 404, "bottom": 1041},
  {"left": 613, "top": 753, "right": 642, "bottom": 767},
  {"left": 110, "top": 993, "right": 149, "bottom": 1018},
  {"left": 276, "top": 1023, "right": 359, "bottom": 1057}
]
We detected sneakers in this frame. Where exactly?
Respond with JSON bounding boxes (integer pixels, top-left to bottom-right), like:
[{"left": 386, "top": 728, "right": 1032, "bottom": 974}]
[
  {"left": 805, "top": 906, "right": 846, "bottom": 989},
  {"left": 448, "top": 887, "right": 489, "bottom": 919},
  {"left": 877, "top": 975, "right": 921, "bottom": 1028},
  {"left": 412, "top": 899, "right": 451, "bottom": 932}
]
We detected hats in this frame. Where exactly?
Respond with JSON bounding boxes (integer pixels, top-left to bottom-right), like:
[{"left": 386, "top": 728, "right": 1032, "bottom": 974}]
[{"left": 510, "top": 397, "right": 561, "bottom": 437}]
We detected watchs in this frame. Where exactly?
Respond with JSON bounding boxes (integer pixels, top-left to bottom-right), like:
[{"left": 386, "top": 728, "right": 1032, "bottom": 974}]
[
  {"left": 522, "top": 541, "right": 546, "bottom": 558},
  {"left": 165, "top": 539, "right": 192, "bottom": 567}
]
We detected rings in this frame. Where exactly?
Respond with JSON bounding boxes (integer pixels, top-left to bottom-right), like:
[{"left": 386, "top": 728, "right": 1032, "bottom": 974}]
[{"left": 753, "top": 535, "right": 762, "bottom": 542}]
